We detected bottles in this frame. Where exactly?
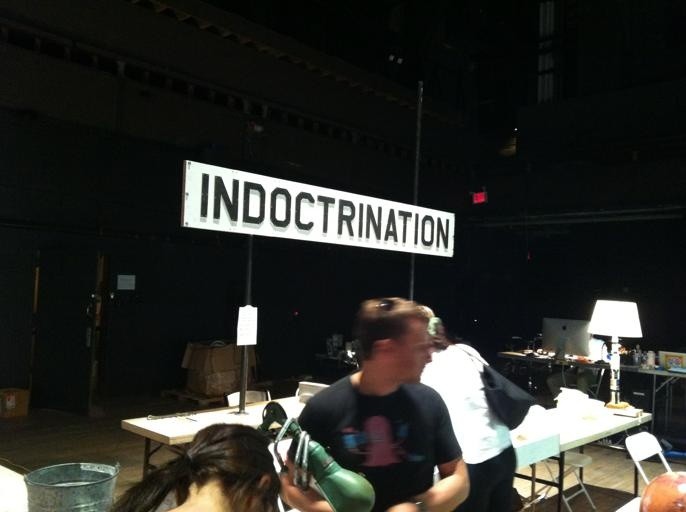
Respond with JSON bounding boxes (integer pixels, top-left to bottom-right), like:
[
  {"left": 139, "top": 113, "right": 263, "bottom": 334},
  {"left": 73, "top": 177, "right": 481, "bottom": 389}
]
[{"left": 647, "top": 350, "right": 656, "bottom": 368}]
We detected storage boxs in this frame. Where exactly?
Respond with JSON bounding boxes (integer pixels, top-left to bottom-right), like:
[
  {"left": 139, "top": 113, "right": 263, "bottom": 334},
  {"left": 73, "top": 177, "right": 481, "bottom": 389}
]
[
  {"left": 185, "top": 343, "right": 253, "bottom": 396},
  {"left": 0, "top": 387, "right": 30, "bottom": 417}
]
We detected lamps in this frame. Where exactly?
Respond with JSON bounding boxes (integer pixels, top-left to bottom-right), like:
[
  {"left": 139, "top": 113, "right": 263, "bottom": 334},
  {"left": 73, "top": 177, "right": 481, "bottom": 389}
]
[
  {"left": 587, "top": 300, "right": 643, "bottom": 408},
  {"left": 257, "top": 402, "right": 375, "bottom": 511}
]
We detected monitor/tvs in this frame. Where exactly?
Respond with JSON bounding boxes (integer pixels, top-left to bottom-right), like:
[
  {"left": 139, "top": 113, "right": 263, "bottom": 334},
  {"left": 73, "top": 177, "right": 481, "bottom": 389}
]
[{"left": 541, "top": 318, "right": 590, "bottom": 360}]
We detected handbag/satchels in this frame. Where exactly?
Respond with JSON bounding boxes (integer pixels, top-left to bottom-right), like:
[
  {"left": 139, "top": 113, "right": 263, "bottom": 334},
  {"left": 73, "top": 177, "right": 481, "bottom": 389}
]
[{"left": 479, "top": 364, "right": 533, "bottom": 430}]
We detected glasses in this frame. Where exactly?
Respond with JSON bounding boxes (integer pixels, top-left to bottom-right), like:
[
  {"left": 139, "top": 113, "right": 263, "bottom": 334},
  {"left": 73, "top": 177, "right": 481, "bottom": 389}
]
[{"left": 379, "top": 299, "right": 394, "bottom": 311}]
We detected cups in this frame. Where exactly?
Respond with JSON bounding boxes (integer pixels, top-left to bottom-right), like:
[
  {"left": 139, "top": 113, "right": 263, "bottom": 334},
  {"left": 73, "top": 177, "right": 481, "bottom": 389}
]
[{"left": 633, "top": 355, "right": 642, "bottom": 366}]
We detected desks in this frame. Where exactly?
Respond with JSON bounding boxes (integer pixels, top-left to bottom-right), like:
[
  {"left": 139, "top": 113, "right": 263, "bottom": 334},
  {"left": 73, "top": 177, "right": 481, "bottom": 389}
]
[
  {"left": 121, "top": 381, "right": 331, "bottom": 481},
  {"left": 498, "top": 351, "right": 686, "bottom": 461},
  {"left": 434, "top": 399, "right": 652, "bottom": 511},
  {"left": 614, "top": 497, "right": 641, "bottom": 512}
]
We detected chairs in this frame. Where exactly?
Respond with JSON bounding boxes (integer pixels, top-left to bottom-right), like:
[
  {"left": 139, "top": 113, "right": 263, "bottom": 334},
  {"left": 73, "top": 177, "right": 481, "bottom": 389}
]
[
  {"left": 223, "top": 389, "right": 272, "bottom": 406},
  {"left": 625, "top": 432, "right": 672, "bottom": 485},
  {"left": 311, "top": 354, "right": 360, "bottom": 385},
  {"left": 540, "top": 451, "right": 596, "bottom": 512}
]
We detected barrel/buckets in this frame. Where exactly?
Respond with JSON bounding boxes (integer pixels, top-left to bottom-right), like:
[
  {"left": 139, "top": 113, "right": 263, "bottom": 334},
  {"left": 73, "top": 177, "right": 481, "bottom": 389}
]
[{"left": 23, "top": 463, "right": 121, "bottom": 512}]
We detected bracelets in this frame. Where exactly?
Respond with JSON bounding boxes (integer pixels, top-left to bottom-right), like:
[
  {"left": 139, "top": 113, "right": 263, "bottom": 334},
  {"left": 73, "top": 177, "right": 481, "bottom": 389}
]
[{"left": 407, "top": 496, "right": 427, "bottom": 511}]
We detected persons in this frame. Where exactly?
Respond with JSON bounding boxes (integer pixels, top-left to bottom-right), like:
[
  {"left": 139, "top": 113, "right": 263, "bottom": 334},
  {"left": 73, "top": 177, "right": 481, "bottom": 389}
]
[
  {"left": 111, "top": 423, "right": 280, "bottom": 511},
  {"left": 277, "top": 296, "right": 472, "bottom": 512},
  {"left": 415, "top": 303, "right": 517, "bottom": 512}
]
